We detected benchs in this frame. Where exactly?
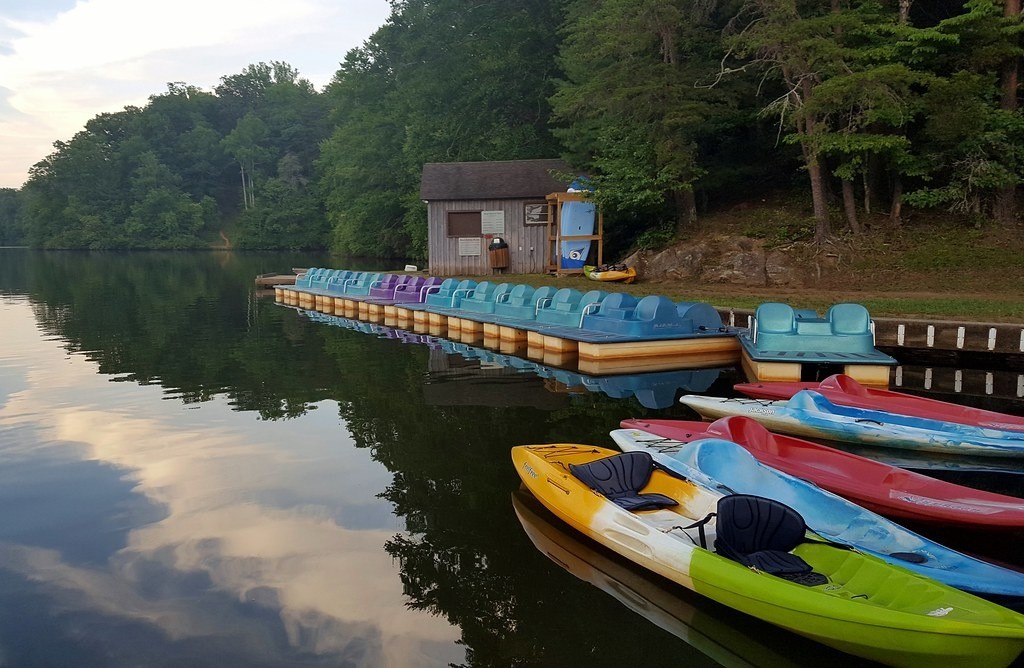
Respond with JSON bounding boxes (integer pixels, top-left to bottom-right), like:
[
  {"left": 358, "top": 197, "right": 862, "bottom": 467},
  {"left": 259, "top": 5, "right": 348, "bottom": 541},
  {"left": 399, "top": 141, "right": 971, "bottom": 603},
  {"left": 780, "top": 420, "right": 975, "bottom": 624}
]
[
  {"left": 463, "top": 281, "right": 514, "bottom": 314},
  {"left": 370, "top": 275, "right": 412, "bottom": 300},
  {"left": 584, "top": 292, "right": 679, "bottom": 337},
  {"left": 329, "top": 271, "right": 362, "bottom": 293},
  {"left": 295, "top": 268, "right": 325, "bottom": 287},
  {"left": 348, "top": 272, "right": 384, "bottom": 295},
  {"left": 495, "top": 285, "right": 557, "bottom": 319},
  {"left": 537, "top": 289, "right": 600, "bottom": 327},
  {"left": 393, "top": 276, "right": 443, "bottom": 306},
  {"left": 313, "top": 270, "right": 341, "bottom": 290},
  {"left": 423, "top": 279, "right": 476, "bottom": 309},
  {"left": 755, "top": 304, "right": 873, "bottom": 351}
]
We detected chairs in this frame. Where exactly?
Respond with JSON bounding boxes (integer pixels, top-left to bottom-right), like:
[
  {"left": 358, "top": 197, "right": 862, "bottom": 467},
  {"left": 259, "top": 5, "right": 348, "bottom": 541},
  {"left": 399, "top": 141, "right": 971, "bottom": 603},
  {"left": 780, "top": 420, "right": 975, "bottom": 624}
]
[
  {"left": 678, "top": 437, "right": 758, "bottom": 482},
  {"left": 708, "top": 416, "right": 777, "bottom": 454},
  {"left": 819, "top": 374, "right": 867, "bottom": 395},
  {"left": 789, "top": 389, "right": 835, "bottom": 414},
  {"left": 716, "top": 494, "right": 814, "bottom": 576},
  {"left": 571, "top": 451, "right": 680, "bottom": 514}
]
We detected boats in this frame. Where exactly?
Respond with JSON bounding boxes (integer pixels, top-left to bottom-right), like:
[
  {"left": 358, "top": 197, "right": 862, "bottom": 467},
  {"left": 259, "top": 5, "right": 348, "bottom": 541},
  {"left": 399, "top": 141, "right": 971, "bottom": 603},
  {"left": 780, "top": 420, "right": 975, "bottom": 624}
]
[
  {"left": 620, "top": 414, "right": 1024, "bottom": 527},
  {"left": 273, "top": 266, "right": 749, "bottom": 358},
  {"left": 733, "top": 372, "right": 1024, "bottom": 432},
  {"left": 737, "top": 301, "right": 899, "bottom": 387},
  {"left": 678, "top": 389, "right": 1024, "bottom": 458},
  {"left": 510, "top": 483, "right": 893, "bottom": 668},
  {"left": 583, "top": 264, "right": 636, "bottom": 281},
  {"left": 556, "top": 176, "right": 595, "bottom": 276},
  {"left": 512, "top": 443, "right": 1024, "bottom": 668},
  {"left": 609, "top": 429, "right": 1024, "bottom": 597},
  {"left": 274, "top": 294, "right": 741, "bottom": 408}
]
